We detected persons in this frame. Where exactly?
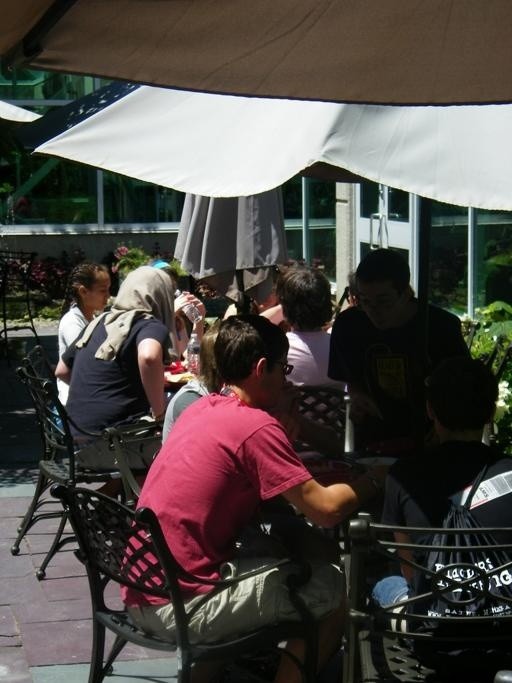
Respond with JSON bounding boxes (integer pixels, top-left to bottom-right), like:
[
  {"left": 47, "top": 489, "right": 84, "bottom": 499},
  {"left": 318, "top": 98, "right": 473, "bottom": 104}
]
[
  {"left": 163, "top": 325, "right": 341, "bottom": 560},
  {"left": 347, "top": 272, "right": 356, "bottom": 306},
  {"left": 162, "top": 268, "right": 207, "bottom": 361},
  {"left": 258, "top": 268, "right": 345, "bottom": 459},
  {"left": 327, "top": 247, "right": 471, "bottom": 607},
  {"left": 52, "top": 263, "right": 111, "bottom": 430},
  {"left": 121, "top": 313, "right": 350, "bottom": 683},
  {"left": 371, "top": 357, "right": 512, "bottom": 683},
  {"left": 55, "top": 267, "right": 175, "bottom": 472},
  {"left": 223, "top": 266, "right": 291, "bottom": 335}
]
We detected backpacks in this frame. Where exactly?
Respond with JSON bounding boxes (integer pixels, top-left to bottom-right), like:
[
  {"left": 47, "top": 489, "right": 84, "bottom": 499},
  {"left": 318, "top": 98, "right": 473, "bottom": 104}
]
[{"left": 414, "top": 446, "right": 512, "bottom": 617}]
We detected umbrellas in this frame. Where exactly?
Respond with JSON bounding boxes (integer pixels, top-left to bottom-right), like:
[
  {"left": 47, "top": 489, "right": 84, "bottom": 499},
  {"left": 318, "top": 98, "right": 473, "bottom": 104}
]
[
  {"left": 0, "top": 0, "right": 512, "bottom": 106},
  {"left": 172, "top": 188, "right": 288, "bottom": 302},
  {"left": 29, "top": 84, "right": 512, "bottom": 267}
]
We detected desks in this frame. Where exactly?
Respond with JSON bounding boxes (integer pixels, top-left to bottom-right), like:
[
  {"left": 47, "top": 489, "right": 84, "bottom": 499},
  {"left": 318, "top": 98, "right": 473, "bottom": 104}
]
[{"left": 0, "top": 249, "right": 47, "bottom": 362}]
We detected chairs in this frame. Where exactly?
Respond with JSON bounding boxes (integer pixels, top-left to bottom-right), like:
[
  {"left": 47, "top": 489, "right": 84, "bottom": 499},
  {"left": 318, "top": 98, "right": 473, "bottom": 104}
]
[
  {"left": 10, "top": 366, "right": 157, "bottom": 580},
  {"left": 23, "top": 345, "right": 53, "bottom": 379},
  {"left": 50, "top": 482, "right": 319, "bottom": 682},
  {"left": 341, "top": 512, "right": 512, "bottom": 683},
  {"left": 296, "top": 384, "right": 347, "bottom": 452},
  {"left": 461, "top": 320, "right": 481, "bottom": 347},
  {"left": 104, "top": 426, "right": 165, "bottom": 503},
  {"left": 486, "top": 336, "right": 512, "bottom": 380}
]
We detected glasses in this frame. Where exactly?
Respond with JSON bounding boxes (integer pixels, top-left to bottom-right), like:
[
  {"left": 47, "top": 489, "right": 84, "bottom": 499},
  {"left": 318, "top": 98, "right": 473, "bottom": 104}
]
[
  {"left": 282, "top": 364, "right": 294, "bottom": 374},
  {"left": 359, "top": 294, "right": 402, "bottom": 313}
]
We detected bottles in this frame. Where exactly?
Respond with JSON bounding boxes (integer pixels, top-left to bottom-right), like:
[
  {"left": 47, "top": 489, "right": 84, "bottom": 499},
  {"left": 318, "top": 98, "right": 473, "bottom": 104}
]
[
  {"left": 173, "top": 288, "right": 202, "bottom": 324},
  {"left": 186, "top": 332, "right": 200, "bottom": 375}
]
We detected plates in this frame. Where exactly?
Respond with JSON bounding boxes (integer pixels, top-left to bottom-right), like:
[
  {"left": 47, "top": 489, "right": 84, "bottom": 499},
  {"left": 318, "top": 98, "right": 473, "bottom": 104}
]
[{"left": 356, "top": 457, "right": 399, "bottom": 467}]
